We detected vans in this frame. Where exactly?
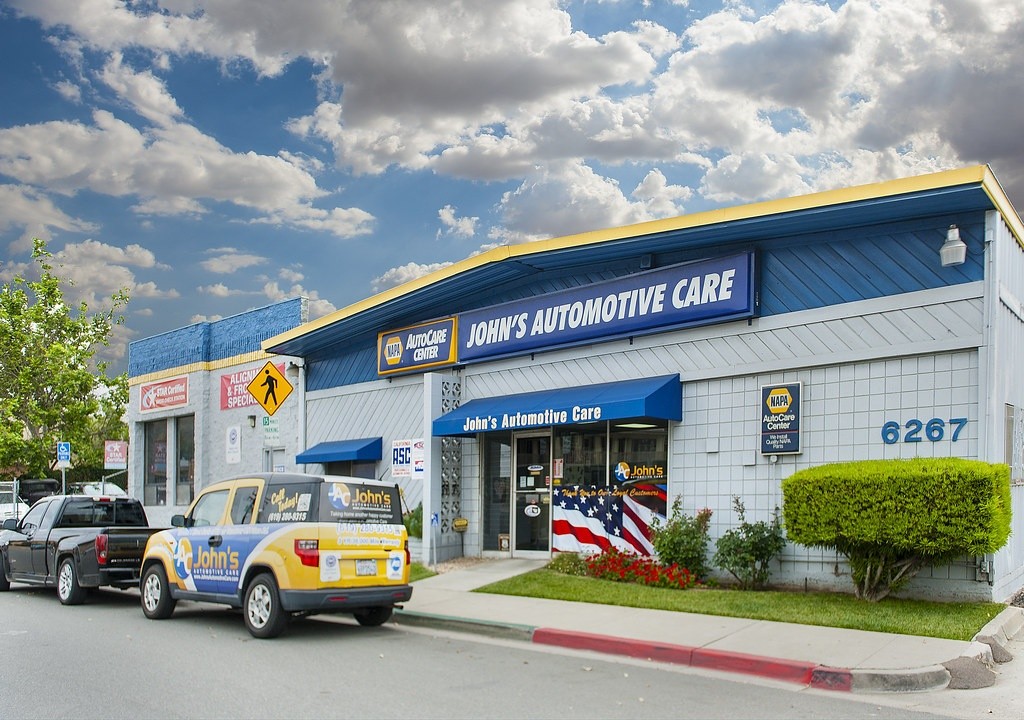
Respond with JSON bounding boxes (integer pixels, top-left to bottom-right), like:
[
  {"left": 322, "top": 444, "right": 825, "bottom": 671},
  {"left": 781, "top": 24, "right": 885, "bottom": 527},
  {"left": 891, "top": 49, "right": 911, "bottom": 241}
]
[{"left": 66, "top": 482, "right": 127, "bottom": 497}]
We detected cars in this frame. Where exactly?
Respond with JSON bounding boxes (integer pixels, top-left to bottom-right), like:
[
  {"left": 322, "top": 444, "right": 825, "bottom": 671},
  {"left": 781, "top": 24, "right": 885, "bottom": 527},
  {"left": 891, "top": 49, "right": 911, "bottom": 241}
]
[
  {"left": 0, "top": 491, "right": 31, "bottom": 526},
  {"left": 138, "top": 471, "right": 414, "bottom": 639},
  {"left": 18, "top": 479, "right": 62, "bottom": 505}
]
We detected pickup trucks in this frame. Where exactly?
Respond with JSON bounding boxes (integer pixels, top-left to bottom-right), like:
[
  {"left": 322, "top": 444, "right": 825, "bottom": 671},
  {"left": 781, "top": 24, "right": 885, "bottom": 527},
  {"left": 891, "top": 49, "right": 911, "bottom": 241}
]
[{"left": 0, "top": 494, "right": 174, "bottom": 605}]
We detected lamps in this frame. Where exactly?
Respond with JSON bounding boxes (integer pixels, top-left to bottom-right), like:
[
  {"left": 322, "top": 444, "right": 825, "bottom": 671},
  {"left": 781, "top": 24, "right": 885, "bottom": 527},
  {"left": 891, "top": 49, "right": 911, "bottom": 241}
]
[
  {"left": 248, "top": 416, "right": 256, "bottom": 427},
  {"left": 286, "top": 362, "right": 305, "bottom": 377},
  {"left": 940, "top": 224, "right": 967, "bottom": 267}
]
